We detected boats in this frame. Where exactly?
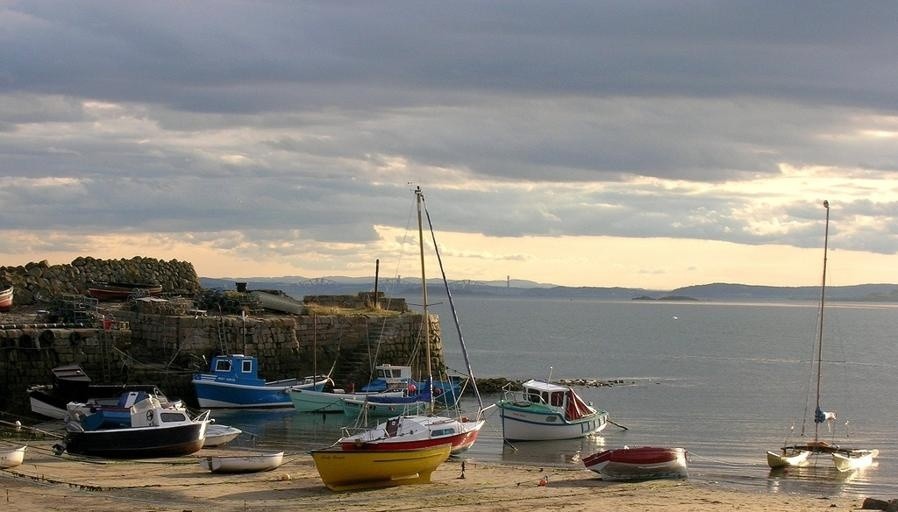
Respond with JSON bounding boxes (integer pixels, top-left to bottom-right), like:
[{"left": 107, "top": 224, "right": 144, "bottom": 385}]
[
  {"left": 193, "top": 353, "right": 329, "bottom": 412},
  {"left": 582, "top": 445, "right": 688, "bottom": 479},
  {"left": 497, "top": 379, "right": 609, "bottom": 443},
  {"left": 0, "top": 444, "right": 28, "bottom": 468},
  {"left": 87, "top": 285, "right": 162, "bottom": 300},
  {"left": 210, "top": 451, "right": 284, "bottom": 474},
  {"left": 0, "top": 285, "right": 14, "bottom": 313},
  {"left": 29, "top": 363, "right": 244, "bottom": 460}
]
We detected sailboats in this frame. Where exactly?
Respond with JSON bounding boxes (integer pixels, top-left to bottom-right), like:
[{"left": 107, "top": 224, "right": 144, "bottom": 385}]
[{"left": 766, "top": 201, "right": 880, "bottom": 473}]
